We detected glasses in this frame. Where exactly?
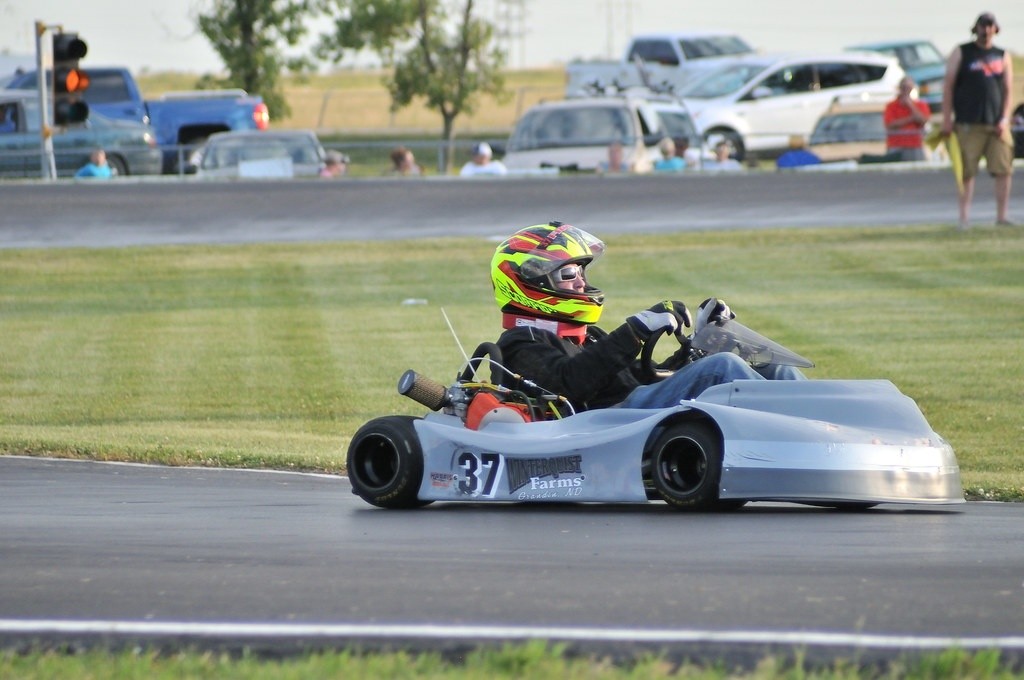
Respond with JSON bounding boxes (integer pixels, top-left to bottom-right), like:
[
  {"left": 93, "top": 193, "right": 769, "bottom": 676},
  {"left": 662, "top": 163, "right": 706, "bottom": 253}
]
[{"left": 552, "top": 264, "right": 585, "bottom": 283}]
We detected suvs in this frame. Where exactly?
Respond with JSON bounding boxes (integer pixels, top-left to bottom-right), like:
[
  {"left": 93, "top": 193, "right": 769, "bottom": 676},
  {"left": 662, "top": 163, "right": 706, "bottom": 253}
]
[{"left": 502, "top": 85, "right": 703, "bottom": 175}]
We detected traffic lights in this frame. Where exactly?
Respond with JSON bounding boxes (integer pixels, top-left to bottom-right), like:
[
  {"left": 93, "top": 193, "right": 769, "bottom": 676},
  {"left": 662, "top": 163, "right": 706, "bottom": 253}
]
[{"left": 50, "top": 33, "right": 92, "bottom": 127}]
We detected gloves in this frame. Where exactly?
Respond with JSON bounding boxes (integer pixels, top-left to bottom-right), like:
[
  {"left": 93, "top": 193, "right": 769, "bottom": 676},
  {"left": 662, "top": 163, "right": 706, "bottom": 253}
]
[
  {"left": 689, "top": 297, "right": 736, "bottom": 340},
  {"left": 626, "top": 300, "right": 692, "bottom": 338}
]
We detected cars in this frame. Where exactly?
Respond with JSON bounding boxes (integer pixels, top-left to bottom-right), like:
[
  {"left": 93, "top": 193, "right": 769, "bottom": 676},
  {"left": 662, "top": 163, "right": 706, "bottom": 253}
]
[
  {"left": 0, "top": 88, "right": 163, "bottom": 177},
  {"left": 171, "top": 130, "right": 349, "bottom": 179},
  {"left": 571, "top": 28, "right": 948, "bottom": 165}
]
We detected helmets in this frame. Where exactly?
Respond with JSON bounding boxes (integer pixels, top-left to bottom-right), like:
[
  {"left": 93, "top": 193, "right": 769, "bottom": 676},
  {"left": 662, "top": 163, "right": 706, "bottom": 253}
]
[{"left": 491, "top": 219, "right": 605, "bottom": 324}]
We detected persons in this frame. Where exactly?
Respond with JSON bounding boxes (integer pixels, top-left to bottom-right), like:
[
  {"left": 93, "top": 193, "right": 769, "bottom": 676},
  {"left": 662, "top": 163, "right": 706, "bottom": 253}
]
[
  {"left": 491, "top": 221, "right": 809, "bottom": 420},
  {"left": 777, "top": 136, "right": 823, "bottom": 167},
  {"left": 75, "top": 150, "right": 113, "bottom": 178},
  {"left": 654, "top": 137, "right": 686, "bottom": 171},
  {"left": 884, "top": 76, "right": 930, "bottom": 160},
  {"left": 943, "top": 12, "right": 1016, "bottom": 226},
  {"left": 460, "top": 142, "right": 505, "bottom": 177},
  {"left": 702, "top": 143, "right": 743, "bottom": 171},
  {"left": 320, "top": 150, "right": 343, "bottom": 177},
  {"left": 597, "top": 142, "right": 627, "bottom": 173},
  {"left": 392, "top": 148, "right": 421, "bottom": 176}
]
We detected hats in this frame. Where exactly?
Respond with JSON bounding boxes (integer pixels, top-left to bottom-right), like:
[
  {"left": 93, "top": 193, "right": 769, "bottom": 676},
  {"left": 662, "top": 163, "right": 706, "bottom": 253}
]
[{"left": 973, "top": 14, "right": 1000, "bottom": 36}]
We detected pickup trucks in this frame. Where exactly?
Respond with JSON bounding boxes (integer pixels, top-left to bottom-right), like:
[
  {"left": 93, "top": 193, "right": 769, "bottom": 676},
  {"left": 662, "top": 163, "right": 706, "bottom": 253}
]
[{"left": 0, "top": 67, "right": 271, "bottom": 174}]
